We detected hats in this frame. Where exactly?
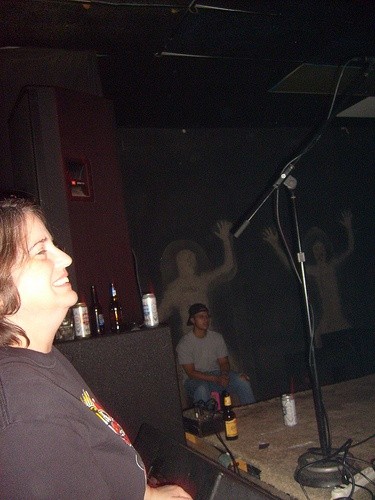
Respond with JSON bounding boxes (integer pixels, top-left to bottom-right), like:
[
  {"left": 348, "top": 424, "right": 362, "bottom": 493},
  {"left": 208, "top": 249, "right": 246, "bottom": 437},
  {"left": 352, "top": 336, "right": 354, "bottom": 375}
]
[{"left": 187, "top": 303, "right": 209, "bottom": 326}]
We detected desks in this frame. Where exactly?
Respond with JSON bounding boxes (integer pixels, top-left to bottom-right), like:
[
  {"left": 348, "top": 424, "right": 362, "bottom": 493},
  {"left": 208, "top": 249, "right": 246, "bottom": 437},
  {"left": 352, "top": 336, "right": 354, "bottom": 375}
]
[
  {"left": 53, "top": 324, "right": 184, "bottom": 446},
  {"left": 174, "top": 373, "right": 375, "bottom": 500}
]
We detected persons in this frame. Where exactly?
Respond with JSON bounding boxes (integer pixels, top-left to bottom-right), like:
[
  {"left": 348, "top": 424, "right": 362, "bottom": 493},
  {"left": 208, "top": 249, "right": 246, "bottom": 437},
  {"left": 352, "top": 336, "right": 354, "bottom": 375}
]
[
  {"left": 0, "top": 195, "right": 193, "bottom": 500},
  {"left": 176, "top": 303, "right": 256, "bottom": 410}
]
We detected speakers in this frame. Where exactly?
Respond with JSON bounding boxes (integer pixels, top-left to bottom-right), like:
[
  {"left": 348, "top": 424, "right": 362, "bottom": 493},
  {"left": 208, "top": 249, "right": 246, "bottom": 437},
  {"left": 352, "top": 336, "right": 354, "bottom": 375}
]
[{"left": 132, "top": 422, "right": 295, "bottom": 500}]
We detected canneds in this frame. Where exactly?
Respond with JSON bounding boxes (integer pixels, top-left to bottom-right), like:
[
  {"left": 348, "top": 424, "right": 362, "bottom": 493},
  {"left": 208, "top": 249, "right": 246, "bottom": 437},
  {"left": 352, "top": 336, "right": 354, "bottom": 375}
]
[
  {"left": 142, "top": 293, "right": 159, "bottom": 328},
  {"left": 282, "top": 394, "right": 297, "bottom": 426},
  {"left": 73, "top": 302, "right": 91, "bottom": 338}
]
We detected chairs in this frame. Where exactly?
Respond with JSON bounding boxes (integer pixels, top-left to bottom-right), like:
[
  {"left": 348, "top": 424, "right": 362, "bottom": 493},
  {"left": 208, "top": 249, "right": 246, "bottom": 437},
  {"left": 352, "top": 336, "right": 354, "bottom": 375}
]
[{"left": 174, "top": 346, "right": 249, "bottom": 433}]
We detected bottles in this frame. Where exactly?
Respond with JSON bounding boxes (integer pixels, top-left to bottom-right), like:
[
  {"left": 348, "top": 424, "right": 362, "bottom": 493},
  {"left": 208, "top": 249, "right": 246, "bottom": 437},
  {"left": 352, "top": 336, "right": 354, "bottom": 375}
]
[
  {"left": 109, "top": 282, "right": 126, "bottom": 334},
  {"left": 90, "top": 285, "right": 106, "bottom": 336},
  {"left": 223, "top": 392, "right": 239, "bottom": 440}
]
